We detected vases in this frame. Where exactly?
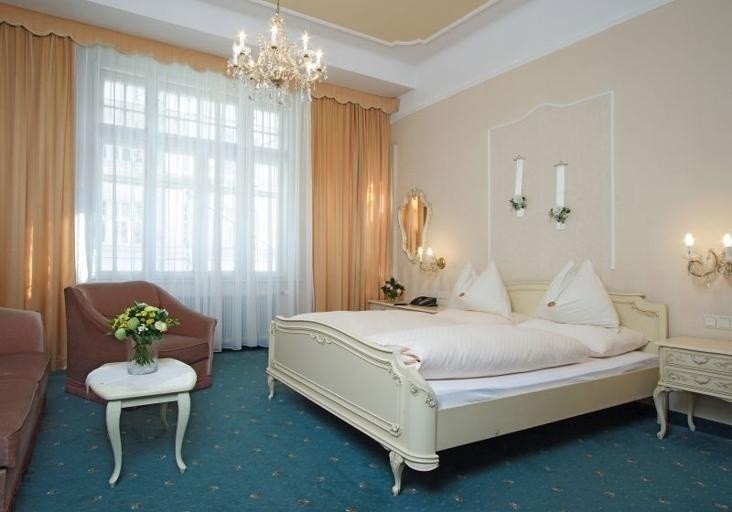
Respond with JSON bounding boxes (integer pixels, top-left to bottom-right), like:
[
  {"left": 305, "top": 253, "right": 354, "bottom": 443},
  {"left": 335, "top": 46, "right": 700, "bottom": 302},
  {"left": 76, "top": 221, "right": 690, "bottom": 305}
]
[
  {"left": 395, "top": 296, "right": 400, "bottom": 302},
  {"left": 124, "top": 339, "right": 159, "bottom": 375}
]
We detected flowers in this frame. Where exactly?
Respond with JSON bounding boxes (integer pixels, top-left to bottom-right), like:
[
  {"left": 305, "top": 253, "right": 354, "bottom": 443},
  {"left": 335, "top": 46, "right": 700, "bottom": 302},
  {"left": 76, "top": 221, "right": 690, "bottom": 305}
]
[
  {"left": 108, "top": 300, "right": 179, "bottom": 365},
  {"left": 381, "top": 273, "right": 405, "bottom": 300}
]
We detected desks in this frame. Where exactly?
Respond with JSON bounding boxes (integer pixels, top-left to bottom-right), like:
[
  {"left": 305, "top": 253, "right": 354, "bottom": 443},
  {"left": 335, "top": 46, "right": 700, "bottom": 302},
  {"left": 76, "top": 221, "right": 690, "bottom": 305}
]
[{"left": 85, "top": 357, "right": 197, "bottom": 486}]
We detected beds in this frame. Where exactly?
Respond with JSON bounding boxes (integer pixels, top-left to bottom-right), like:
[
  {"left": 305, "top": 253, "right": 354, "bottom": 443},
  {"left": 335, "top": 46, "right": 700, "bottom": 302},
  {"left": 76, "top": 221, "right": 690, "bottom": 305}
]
[{"left": 265, "top": 282, "right": 670, "bottom": 498}]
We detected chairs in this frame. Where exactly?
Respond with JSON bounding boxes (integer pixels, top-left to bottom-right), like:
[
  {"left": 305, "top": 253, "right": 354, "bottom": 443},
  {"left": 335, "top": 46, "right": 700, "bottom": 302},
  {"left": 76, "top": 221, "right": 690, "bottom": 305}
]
[{"left": 63, "top": 281, "right": 217, "bottom": 410}]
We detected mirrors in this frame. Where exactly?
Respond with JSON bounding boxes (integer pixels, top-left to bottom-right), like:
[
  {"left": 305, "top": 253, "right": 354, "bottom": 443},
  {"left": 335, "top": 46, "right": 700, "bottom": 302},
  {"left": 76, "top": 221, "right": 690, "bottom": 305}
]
[{"left": 397, "top": 185, "right": 430, "bottom": 263}]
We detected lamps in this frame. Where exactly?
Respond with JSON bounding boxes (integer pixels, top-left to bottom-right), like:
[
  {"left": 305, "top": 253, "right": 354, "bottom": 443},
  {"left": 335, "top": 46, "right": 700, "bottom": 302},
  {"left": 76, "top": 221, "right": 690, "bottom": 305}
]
[
  {"left": 418, "top": 247, "right": 445, "bottom": 271},
  {"left": 226, "top": 0, "right": 328, "bottom": 105},
  {"left": 684, "top": 233, "right": 732, "bottom": 289}
]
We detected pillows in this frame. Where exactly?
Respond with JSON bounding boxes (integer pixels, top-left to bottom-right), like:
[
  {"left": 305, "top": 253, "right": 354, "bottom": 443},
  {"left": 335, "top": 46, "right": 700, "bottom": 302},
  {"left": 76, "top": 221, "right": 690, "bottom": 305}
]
[
  {"left": 446, "top": 260, "right": 512, "bottom": 316},
  {"left": 536, "top": 260, "right": 620, "bottom": 328},
  {"left": 519, "top": 320, "right": 650, "bottom": 357}
]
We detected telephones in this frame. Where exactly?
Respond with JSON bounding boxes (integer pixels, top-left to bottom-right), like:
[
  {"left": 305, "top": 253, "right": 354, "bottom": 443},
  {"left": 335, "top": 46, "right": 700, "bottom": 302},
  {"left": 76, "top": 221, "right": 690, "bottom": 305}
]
[{"left": 411, "top": 296, "right": 438, "bottom": 307}]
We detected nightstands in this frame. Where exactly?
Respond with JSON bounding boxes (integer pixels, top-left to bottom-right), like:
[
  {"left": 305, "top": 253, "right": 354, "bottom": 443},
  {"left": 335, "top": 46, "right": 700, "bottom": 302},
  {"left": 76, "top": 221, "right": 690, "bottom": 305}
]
[
  {"left": 653, "top": 336, "right": 732, "bottom": 440},
  {"left": 368, "top": 299, "right": 444, "bottom": 314}
]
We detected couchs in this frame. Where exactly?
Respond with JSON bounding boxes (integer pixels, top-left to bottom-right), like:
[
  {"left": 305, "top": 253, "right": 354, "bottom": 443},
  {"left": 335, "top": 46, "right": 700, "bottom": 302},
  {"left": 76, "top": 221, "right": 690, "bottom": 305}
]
[{"left": 0, "top": 306, "right": 52, "bottom": 512}]
